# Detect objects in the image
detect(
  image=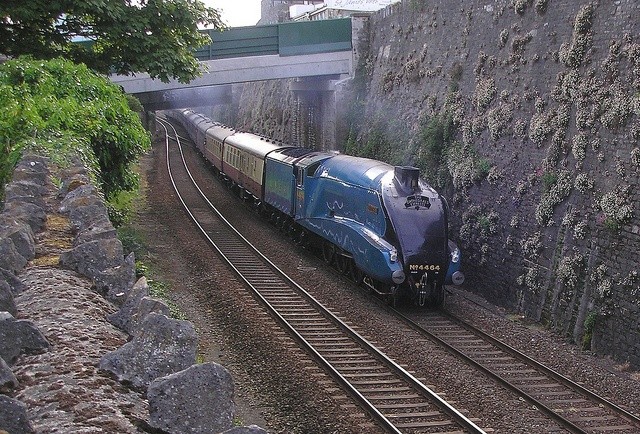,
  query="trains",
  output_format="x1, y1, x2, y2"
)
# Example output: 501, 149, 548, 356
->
155, 108, 465, 309
